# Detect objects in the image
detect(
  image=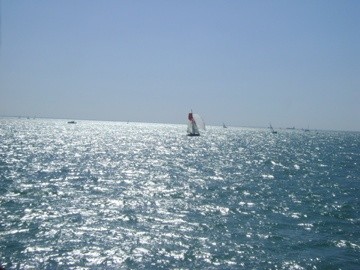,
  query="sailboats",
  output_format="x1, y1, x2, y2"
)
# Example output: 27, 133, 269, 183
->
67, 121, 77, 124
269, 123, 277, 134
223, 122, 227, 128
186, 109, 200, 136
